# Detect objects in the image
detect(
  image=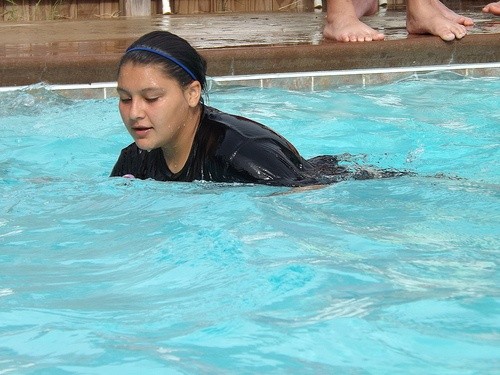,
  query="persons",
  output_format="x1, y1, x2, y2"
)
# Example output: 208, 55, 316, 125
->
109, 31, 306, 182
323, 0, 500, 42
